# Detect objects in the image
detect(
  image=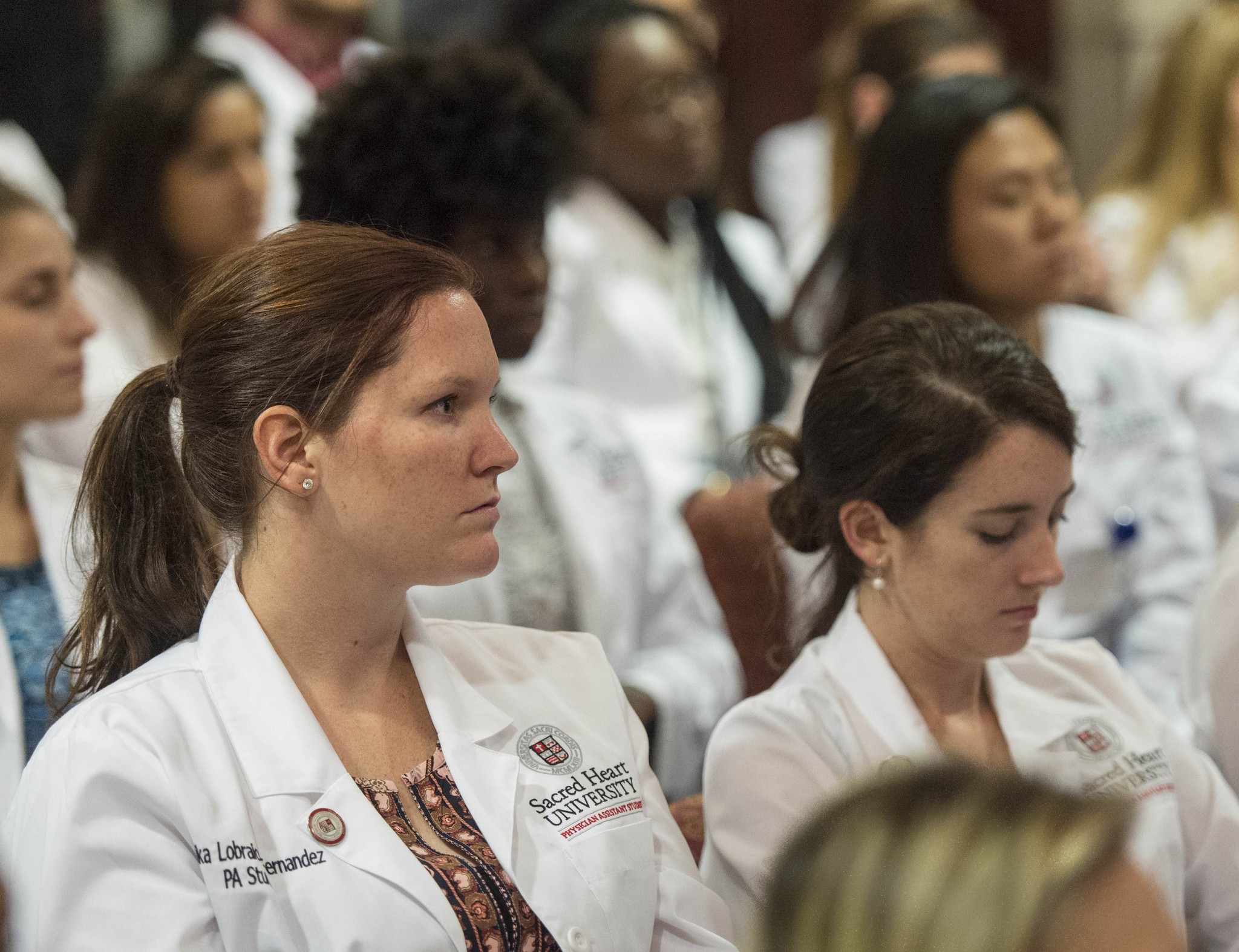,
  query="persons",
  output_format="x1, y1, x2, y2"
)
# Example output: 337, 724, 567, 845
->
1, 218, 695, 952
748, 757, 1191, 952
0, 0, 1239, 864
699, 300, 1239, 952
